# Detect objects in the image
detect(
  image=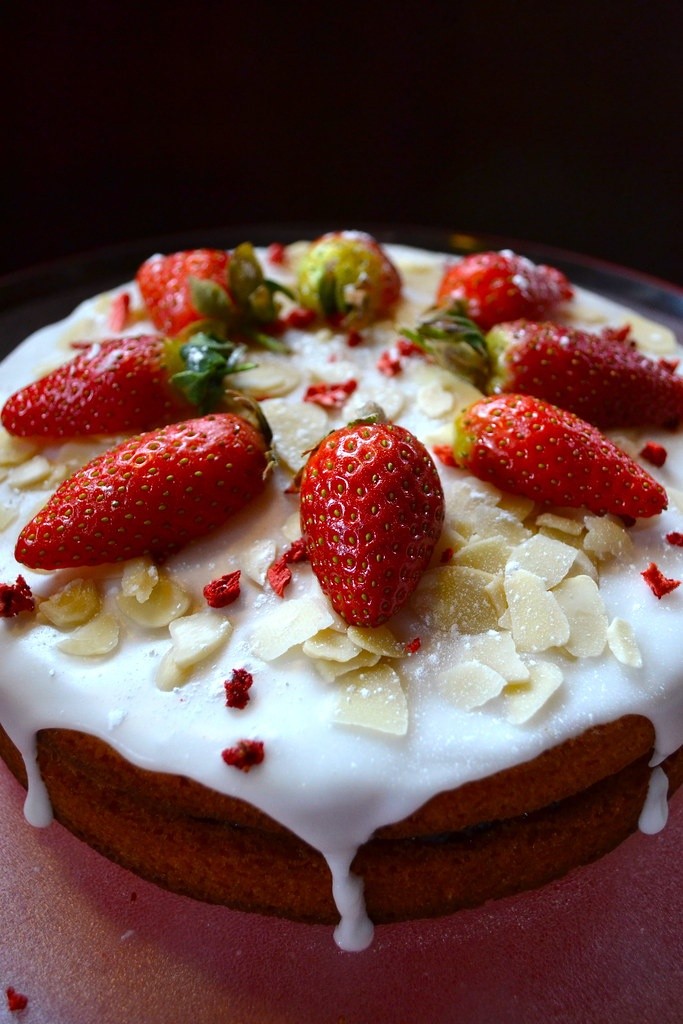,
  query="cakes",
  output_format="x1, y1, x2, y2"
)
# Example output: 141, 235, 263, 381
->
0, 231, 682, 1024
